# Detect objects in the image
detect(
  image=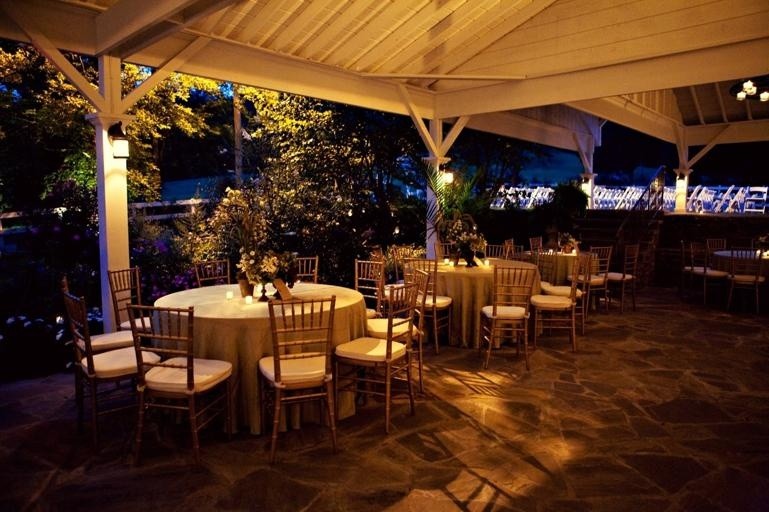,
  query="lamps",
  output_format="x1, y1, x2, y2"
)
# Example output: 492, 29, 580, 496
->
727, 76, 769, 103
438, 165, 459, 186
105, 120, 134, 163
